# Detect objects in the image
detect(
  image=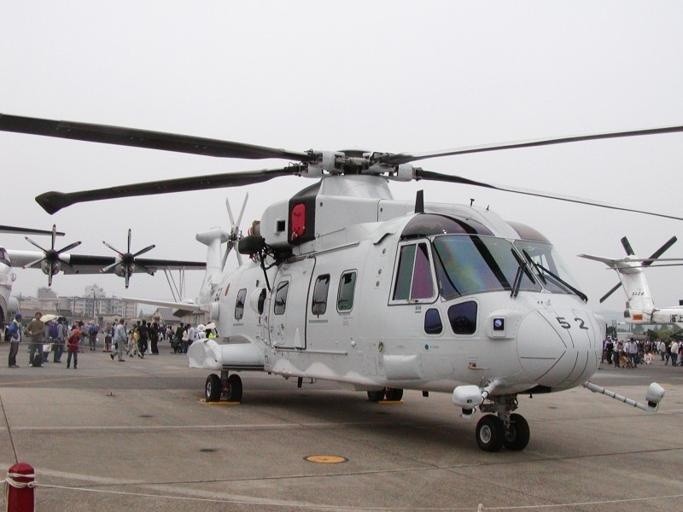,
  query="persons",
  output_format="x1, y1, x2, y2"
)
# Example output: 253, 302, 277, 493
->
4, 311, 221, 369
603, 336, 683, 368
